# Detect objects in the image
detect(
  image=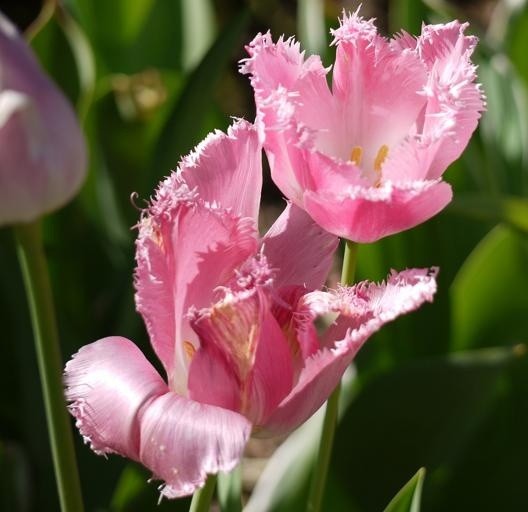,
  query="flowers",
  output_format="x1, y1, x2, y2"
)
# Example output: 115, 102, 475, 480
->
238, 2, 487, 248
62, 116, 439, 512
1, 15, 89, 227
